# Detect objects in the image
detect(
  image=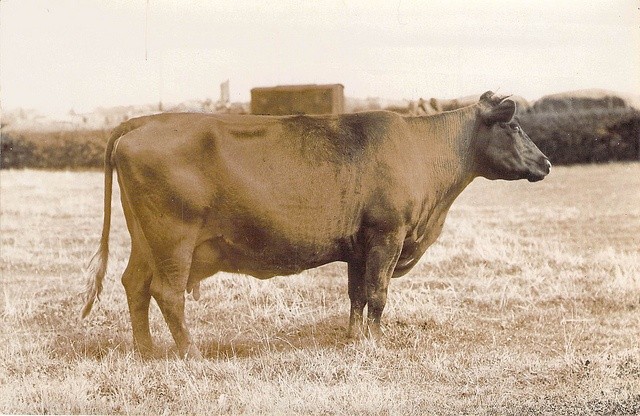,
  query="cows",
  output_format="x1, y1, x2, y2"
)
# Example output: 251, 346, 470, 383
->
80, 90, 554, 362
